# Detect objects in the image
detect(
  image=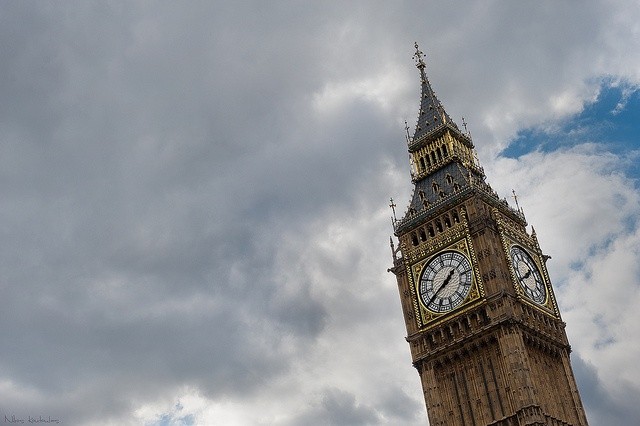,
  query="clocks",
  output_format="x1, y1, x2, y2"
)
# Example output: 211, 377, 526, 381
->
402, 229, 485, 332
495, 206, 561, 320
